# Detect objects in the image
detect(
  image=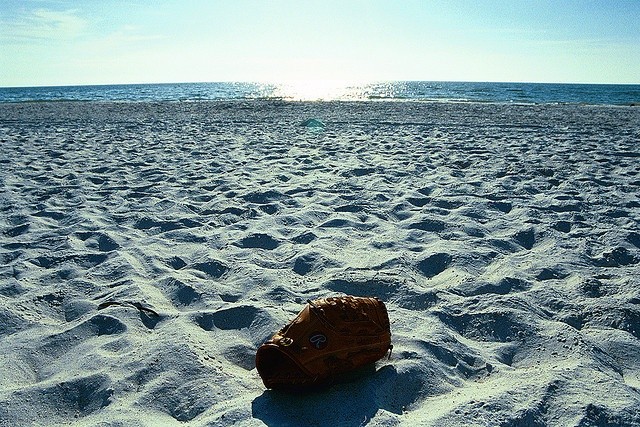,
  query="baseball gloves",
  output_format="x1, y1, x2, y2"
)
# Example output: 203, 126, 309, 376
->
256, 295, 393, 389
